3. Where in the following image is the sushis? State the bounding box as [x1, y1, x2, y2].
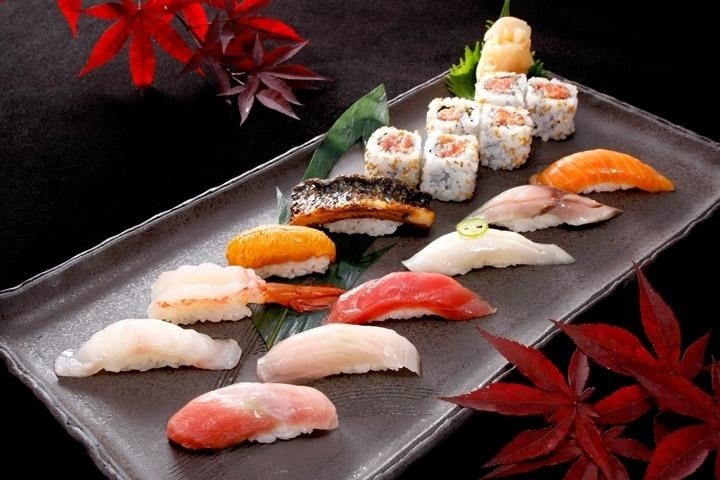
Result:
[363, 70, 578, 203]
